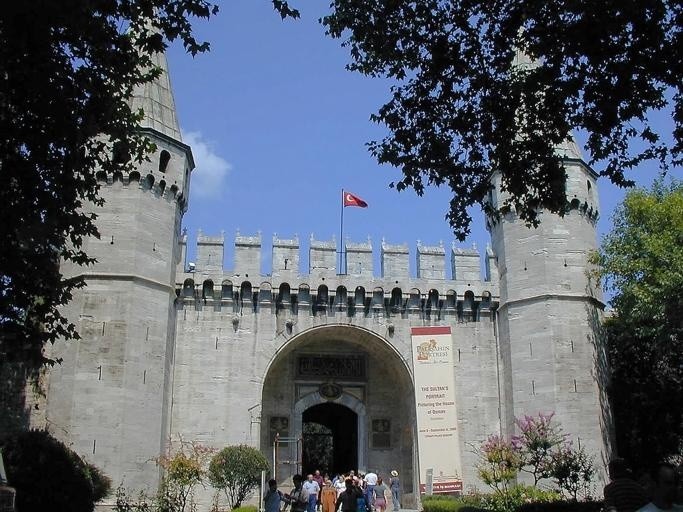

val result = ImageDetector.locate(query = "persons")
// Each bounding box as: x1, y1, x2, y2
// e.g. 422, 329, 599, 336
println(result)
262, 478, 291, 512
388, 470, 401, 512
279, 469, 387, 512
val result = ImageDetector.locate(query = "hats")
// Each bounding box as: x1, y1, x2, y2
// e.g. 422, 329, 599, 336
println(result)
391, 470, 398, 476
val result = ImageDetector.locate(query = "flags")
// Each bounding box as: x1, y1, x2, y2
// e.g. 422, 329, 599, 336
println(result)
341, 188, 369, 211
